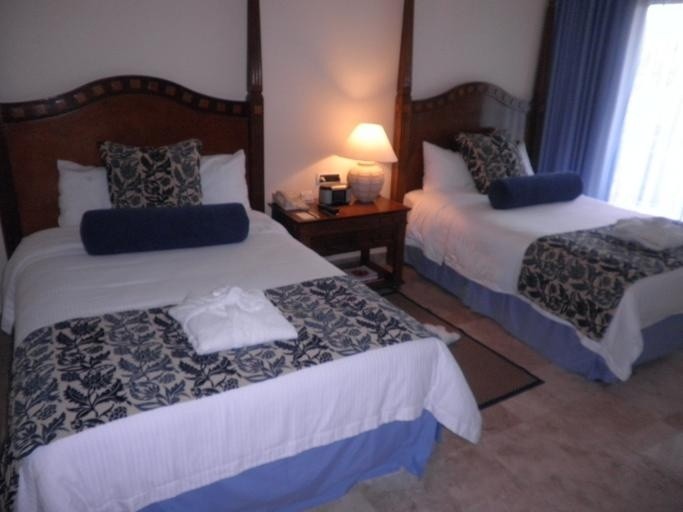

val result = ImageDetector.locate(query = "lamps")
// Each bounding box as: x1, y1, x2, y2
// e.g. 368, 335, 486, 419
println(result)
335, 120, 400, 208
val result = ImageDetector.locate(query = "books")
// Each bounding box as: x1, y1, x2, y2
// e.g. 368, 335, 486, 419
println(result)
343, 264, 384, 282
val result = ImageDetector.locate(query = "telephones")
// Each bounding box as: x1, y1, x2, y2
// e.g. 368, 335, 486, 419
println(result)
272, 190, 310, 211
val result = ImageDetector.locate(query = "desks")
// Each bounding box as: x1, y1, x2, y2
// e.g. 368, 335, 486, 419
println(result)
267, 188, 409, 276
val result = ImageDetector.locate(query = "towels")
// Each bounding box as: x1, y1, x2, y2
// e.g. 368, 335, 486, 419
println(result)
608, 214, 682, 255
166, 287, 295, 352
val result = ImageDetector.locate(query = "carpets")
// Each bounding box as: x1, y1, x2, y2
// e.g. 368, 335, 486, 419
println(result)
336, 261, 547, 418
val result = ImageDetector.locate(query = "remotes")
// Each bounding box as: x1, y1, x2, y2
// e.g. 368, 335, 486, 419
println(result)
319, 203, 339, 214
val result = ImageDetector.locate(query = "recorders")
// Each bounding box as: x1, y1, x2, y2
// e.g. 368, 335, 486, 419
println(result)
319, 184, 351, 205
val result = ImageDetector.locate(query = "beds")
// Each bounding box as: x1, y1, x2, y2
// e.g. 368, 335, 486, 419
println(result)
385, 0, 680, 383
1, 0, 485, 511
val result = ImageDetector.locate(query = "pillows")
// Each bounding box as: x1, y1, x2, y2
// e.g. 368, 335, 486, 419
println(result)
457, 123, 528, 195
79, 196, 254, 257
96, 135, 206, 209
417, 137, 533, 198
486, 167, 583, 207
52, 149, 251, 226
